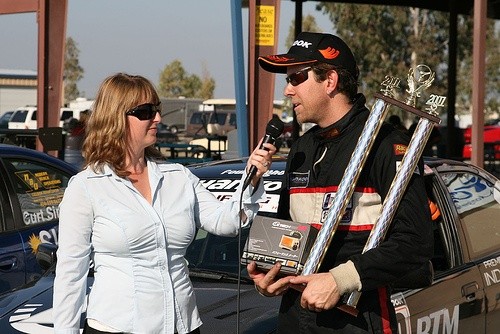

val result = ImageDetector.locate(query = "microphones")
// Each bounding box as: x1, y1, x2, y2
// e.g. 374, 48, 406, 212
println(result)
242, 117, 285, 191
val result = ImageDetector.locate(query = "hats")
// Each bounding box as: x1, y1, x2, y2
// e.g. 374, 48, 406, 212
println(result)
257, 31, 360, 77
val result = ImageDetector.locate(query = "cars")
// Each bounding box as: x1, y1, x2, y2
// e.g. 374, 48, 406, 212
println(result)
0, 154, 500, 334
0, 144, 81, 293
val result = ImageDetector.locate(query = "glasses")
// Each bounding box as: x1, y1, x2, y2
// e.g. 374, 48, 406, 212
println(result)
126, 100, 163, 122
285, 67, 314, 87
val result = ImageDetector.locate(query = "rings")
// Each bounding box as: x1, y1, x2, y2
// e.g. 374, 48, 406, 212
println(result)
264, 161, 270, 167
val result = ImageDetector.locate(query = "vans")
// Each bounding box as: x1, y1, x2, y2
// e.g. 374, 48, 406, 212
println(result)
8, 106, 75, 129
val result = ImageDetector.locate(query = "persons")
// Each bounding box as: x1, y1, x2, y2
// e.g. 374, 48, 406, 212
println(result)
247, 32, 436, 334
389, 116, 408, 135
53, 72, 277, 334
